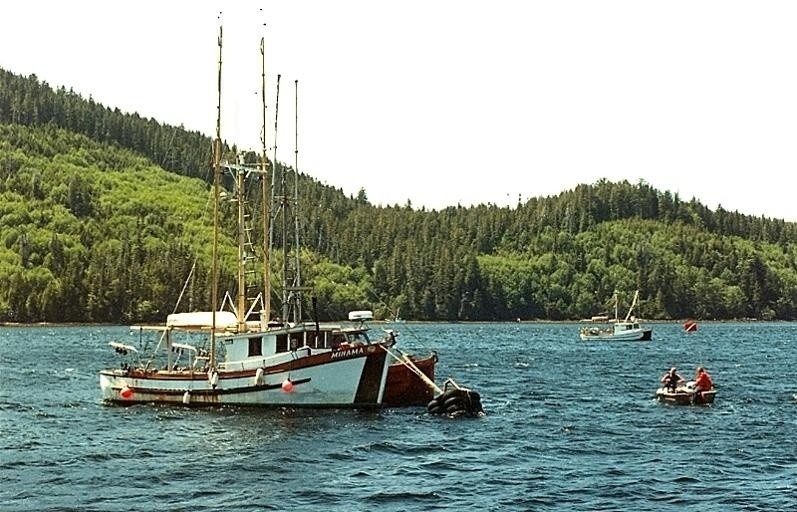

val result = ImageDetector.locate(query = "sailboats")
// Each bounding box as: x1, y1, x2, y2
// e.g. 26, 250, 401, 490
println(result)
578, 289, 653, 341
98, 22, 396, 410
268, 75, 439, 404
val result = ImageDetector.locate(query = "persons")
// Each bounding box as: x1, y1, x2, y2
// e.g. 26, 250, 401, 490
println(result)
291, 330, 342, 349
660, 367, 684, 393
692, 367, 712, 390
581, 325, 612, 336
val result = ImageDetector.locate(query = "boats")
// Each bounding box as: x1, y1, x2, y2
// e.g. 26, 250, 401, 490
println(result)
655, 379, 717, 405
